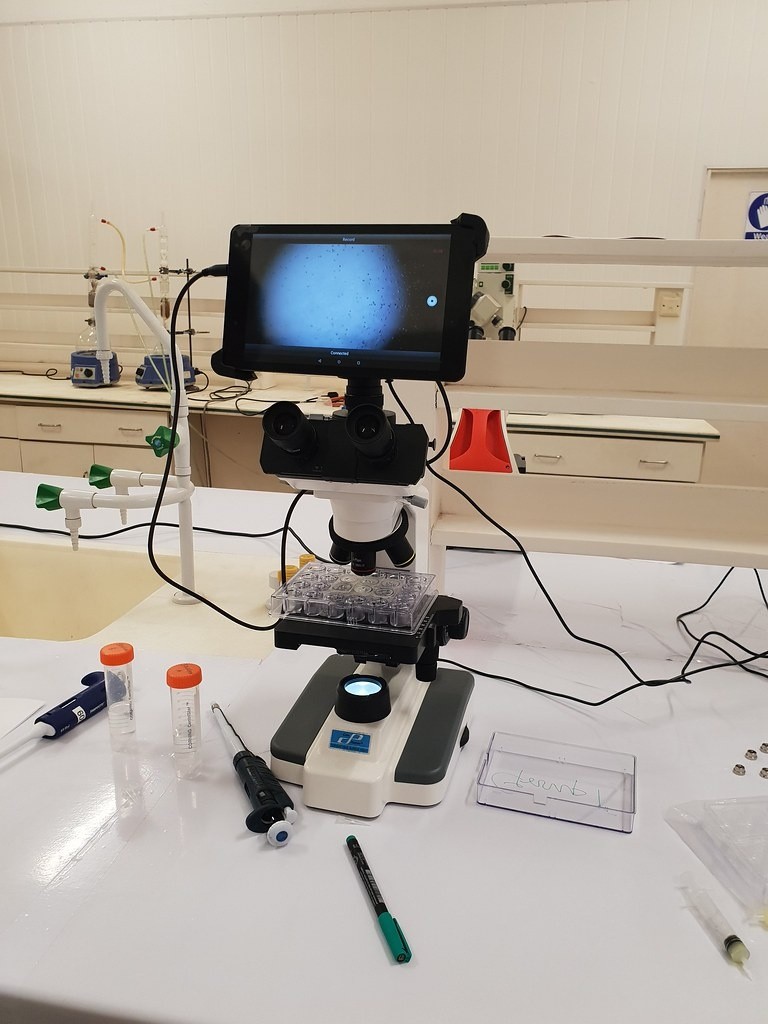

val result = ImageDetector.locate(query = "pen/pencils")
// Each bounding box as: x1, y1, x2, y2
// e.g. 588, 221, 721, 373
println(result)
347, 835, 412, 966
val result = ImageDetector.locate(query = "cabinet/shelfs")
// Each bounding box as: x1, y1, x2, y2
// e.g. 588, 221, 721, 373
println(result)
507, 412, 721, 484
0, 375, 204, 485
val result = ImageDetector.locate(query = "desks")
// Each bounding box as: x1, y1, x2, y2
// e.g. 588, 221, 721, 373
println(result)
0, 471, 768, 1024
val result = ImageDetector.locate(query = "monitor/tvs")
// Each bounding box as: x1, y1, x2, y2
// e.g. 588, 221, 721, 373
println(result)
221, 224, 475, 382
